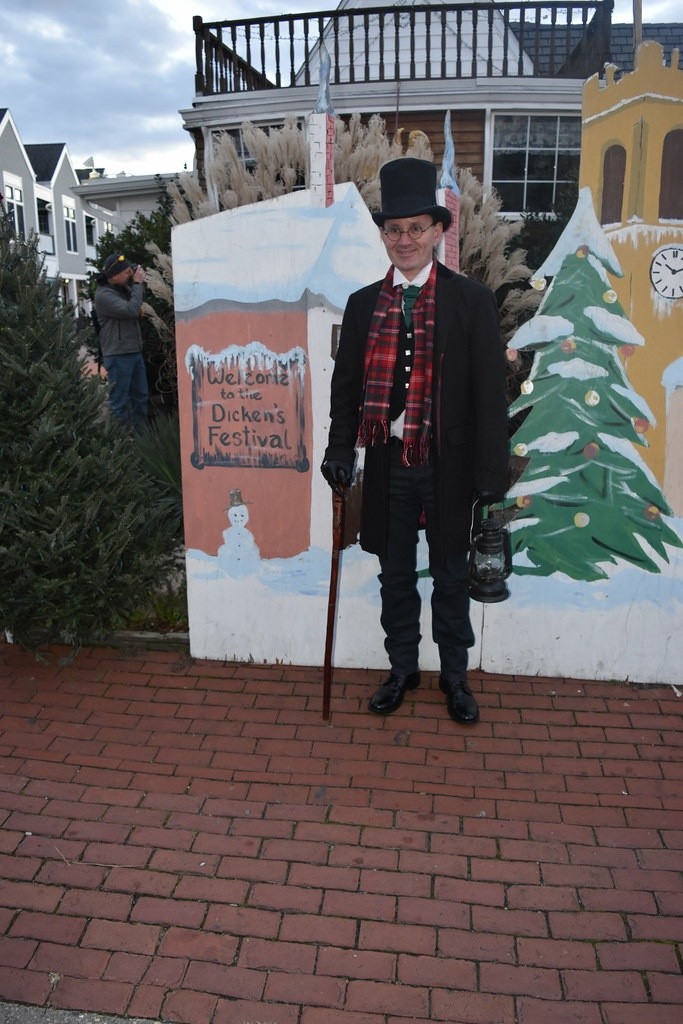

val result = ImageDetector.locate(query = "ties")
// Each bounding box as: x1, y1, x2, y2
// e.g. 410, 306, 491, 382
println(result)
402, 285, 424, 327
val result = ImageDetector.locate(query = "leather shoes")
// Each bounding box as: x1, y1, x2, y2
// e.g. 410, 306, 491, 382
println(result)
367, 673, 420, 715
439, 675, 480, 724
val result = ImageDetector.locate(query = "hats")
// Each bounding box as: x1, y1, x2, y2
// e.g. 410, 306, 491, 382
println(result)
102, 254, 130, 278
372, 158, 453, 232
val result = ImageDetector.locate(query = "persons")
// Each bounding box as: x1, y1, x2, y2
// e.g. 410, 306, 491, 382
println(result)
321, 155, 512, 724
94, 253, 149, 436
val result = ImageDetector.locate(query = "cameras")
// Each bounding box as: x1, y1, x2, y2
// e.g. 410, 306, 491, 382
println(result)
130, 262, 146, 275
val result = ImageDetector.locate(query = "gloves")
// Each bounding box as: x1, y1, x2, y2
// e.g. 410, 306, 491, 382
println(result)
321, 460, 353, 496
476, 489, 505, 508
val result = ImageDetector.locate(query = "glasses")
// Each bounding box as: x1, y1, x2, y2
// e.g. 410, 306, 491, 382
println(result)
381, 222, 436, 242
107, 254, 125, 272
